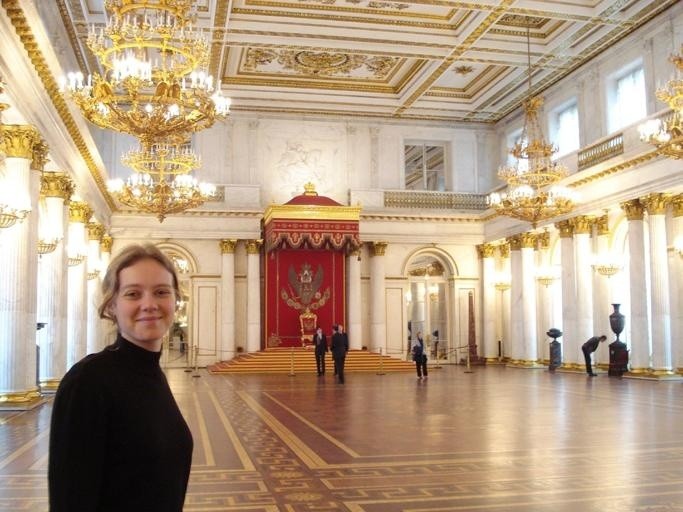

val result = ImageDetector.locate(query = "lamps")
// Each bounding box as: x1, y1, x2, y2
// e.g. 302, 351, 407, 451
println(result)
100, 173, 222, 223
115, 137, 198, 178
51, 66, 235, 147
482, 13, 578, 231
71, 1, 208, 79
637, 44, 683, 162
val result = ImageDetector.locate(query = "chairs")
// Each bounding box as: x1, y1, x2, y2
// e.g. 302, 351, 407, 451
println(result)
297, 308, 321, 348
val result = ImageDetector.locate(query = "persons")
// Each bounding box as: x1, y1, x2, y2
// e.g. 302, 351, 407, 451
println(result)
313, 323, 349, 384
49, 244, 194, 512
582, 335, 607, 376
411, 332, 428, 378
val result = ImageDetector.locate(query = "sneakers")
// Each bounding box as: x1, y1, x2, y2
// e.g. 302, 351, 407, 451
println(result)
587, 372, 598, 377
415, 375, 429, 382
